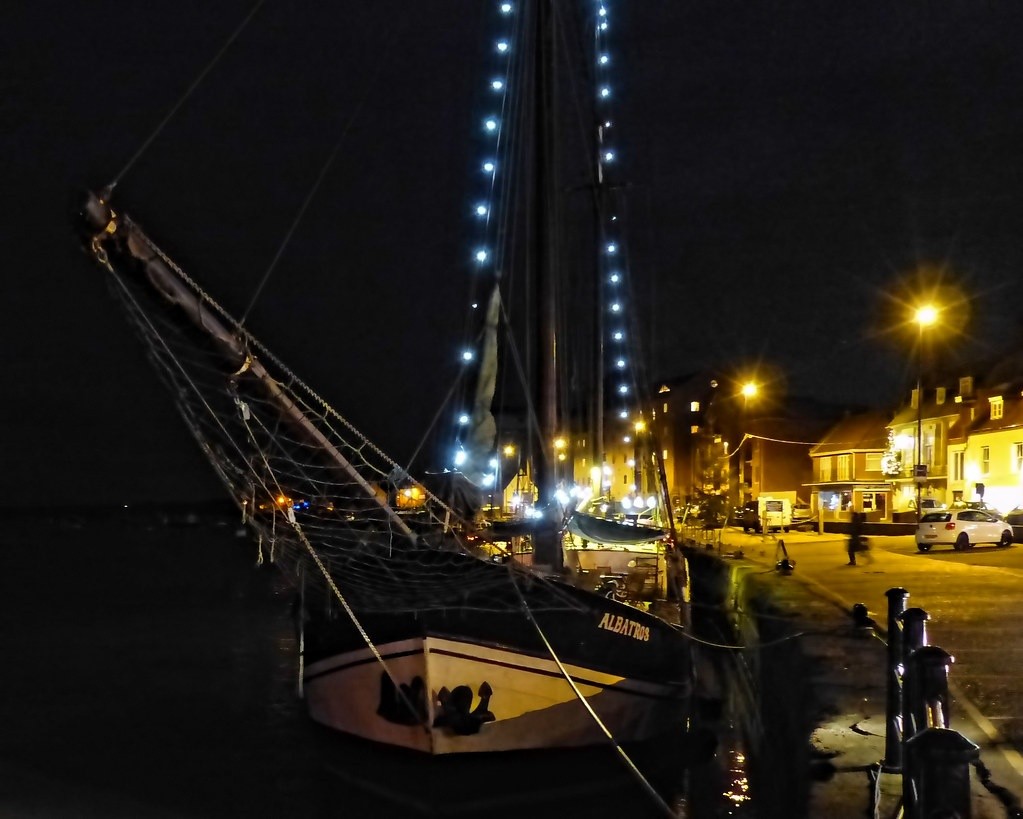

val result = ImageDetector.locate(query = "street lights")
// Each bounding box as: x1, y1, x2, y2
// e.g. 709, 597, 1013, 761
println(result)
742, 384, 757, 433
915, 309, 937, 522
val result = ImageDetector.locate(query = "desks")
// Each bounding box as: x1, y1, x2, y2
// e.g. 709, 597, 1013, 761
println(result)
612, 572, 628, 576
600, 575, 624, 579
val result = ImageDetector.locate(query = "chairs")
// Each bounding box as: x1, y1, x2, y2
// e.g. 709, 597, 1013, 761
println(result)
597, 566, 611, 576
635, 556, 659, 583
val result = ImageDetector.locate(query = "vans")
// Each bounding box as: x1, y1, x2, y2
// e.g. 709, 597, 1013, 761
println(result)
742, 497, 793, 533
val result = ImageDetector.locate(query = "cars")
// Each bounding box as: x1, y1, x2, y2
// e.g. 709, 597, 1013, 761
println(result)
914, 508, 1014, 551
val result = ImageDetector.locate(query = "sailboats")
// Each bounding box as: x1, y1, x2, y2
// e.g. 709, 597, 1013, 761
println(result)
77, 0, 700, 819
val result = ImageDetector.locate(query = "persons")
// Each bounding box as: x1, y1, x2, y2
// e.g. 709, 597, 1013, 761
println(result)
416, 536, 430, 549
437, 531, 463, 552
844, 508, 869, 566
876, 494, 885, 518
949, 494, 966, 509
